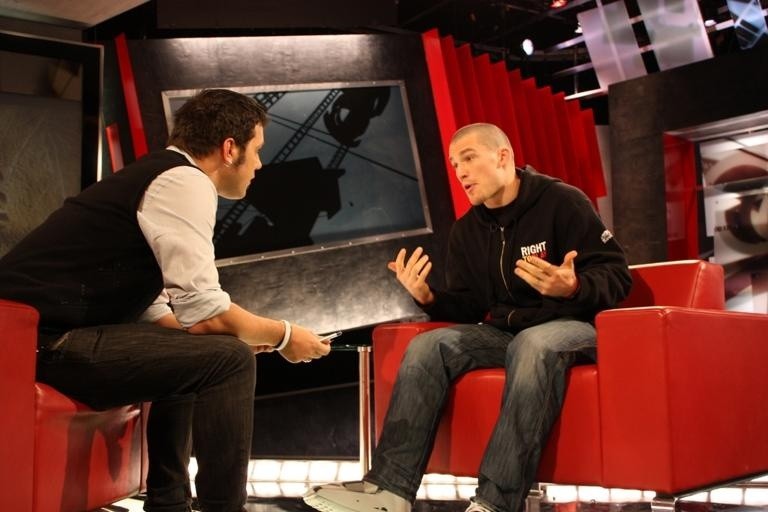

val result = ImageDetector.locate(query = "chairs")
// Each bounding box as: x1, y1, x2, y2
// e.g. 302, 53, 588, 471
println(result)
1, 299, 152, 511
373, 260, 768, 511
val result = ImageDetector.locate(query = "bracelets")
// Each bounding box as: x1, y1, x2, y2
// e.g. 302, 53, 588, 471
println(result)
272, 318, 291, 351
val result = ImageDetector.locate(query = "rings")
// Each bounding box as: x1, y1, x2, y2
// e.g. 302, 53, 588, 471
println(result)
304, 359, 312, 363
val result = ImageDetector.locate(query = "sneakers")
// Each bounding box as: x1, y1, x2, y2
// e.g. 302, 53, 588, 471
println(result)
303, 479, 412, 512
465, 500, 491, 512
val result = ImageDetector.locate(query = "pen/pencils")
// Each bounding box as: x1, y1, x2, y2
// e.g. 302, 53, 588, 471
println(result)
321, 332, 343, 342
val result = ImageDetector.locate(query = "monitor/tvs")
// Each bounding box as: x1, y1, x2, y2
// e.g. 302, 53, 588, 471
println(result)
0, 29, 106, 263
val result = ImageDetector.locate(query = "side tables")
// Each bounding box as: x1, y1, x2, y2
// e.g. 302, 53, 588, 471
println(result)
328, 342, 374, 482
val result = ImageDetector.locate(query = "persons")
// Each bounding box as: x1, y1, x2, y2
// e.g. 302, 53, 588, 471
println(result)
293, 122, 632, 512
0, 86, 333, 512
